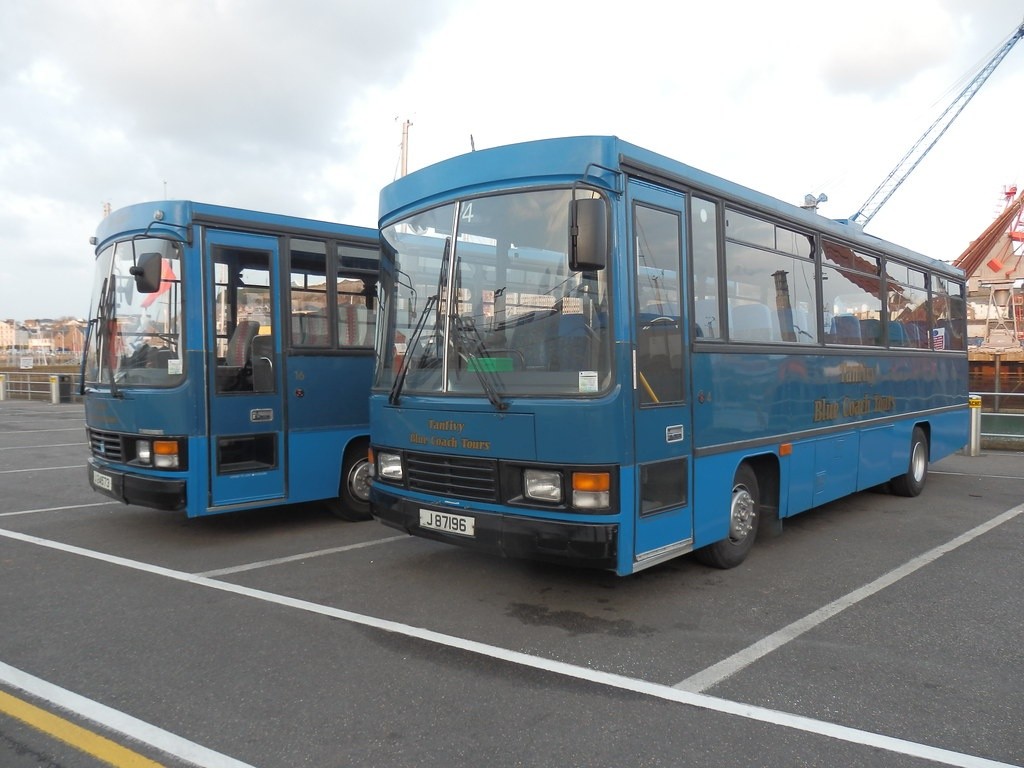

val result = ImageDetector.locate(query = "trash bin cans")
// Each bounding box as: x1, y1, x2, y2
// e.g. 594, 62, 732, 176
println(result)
48, 375, 72, 403
967, 395, 982, 458
0, 374, 7, 400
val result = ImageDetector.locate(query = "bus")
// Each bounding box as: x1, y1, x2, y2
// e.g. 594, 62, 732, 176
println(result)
78, 199, 736, 521
364, 134, 971, 578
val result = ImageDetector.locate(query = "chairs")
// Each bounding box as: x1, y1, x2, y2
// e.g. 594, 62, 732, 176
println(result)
290, 303, 377, 343
510, 311, 589, 368
219, 319, 261, 371
693, 300, 966, 348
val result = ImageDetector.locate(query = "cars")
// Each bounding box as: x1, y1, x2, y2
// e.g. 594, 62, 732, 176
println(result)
5, 347, 70, 356
